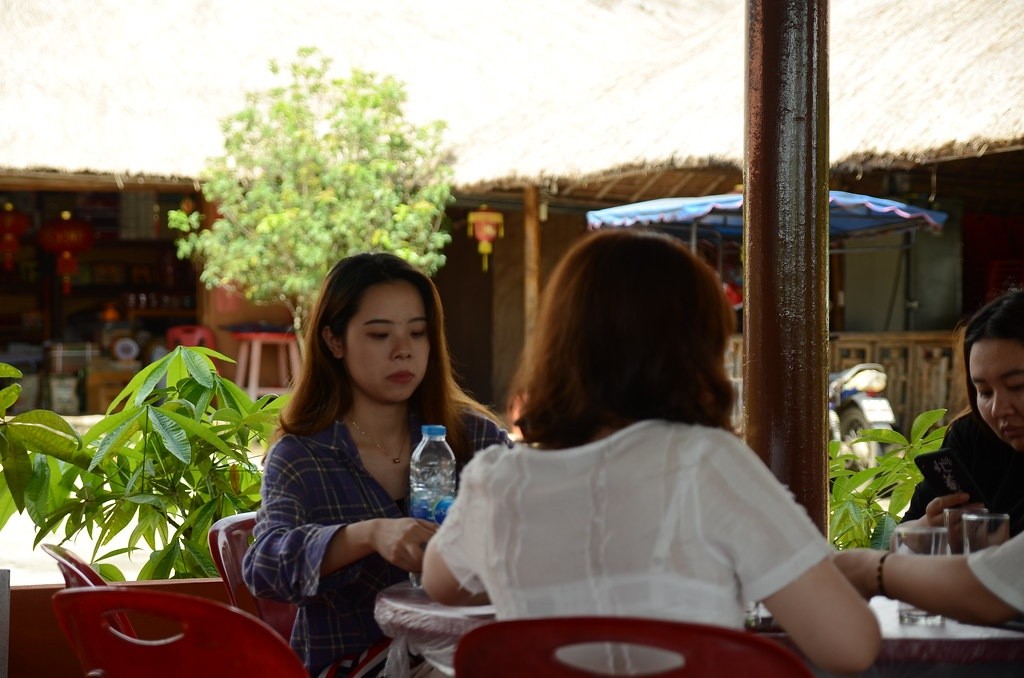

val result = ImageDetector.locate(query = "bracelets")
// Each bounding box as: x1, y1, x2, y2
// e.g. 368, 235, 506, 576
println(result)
876, 553, 890, 594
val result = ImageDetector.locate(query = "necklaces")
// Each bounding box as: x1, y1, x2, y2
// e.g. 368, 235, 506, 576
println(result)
350, 416, 409, 464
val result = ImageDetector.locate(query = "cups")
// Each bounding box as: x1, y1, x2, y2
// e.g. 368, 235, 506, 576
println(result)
896, 526, 948, 627
943, 509, 989, 556
961, 514, 1010, 555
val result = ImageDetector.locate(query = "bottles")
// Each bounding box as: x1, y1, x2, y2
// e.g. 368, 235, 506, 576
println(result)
409, 425, 457, 593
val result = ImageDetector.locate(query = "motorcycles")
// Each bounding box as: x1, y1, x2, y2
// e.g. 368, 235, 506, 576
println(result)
727, 333, 897, 495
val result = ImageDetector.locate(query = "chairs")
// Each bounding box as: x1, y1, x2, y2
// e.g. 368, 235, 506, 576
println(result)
51, 587, 310, 678
208, 511, 300, 644
40, 544, 138, 641
452, 615, 815, 678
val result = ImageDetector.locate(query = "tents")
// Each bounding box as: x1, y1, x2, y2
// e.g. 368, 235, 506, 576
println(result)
588, 184, 946, 331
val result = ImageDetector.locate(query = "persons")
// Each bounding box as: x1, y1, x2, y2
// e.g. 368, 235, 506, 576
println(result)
831, 289, 1024, 627
240, 253, 513, 678
423, 230, 879, 678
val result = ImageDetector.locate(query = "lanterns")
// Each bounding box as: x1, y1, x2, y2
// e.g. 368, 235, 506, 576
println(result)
40, 218, 94, 294
0, 213, 33, 269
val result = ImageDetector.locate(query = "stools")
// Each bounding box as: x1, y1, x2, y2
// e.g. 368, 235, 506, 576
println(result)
230, 333, 301, 404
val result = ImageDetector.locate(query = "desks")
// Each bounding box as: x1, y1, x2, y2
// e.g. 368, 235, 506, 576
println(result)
374, 576, 1024, 678
